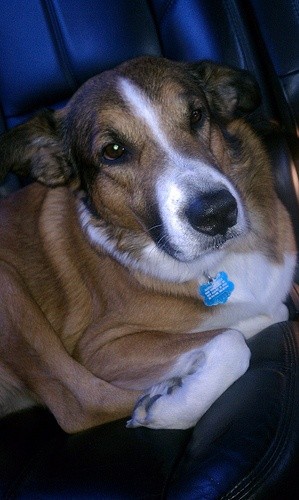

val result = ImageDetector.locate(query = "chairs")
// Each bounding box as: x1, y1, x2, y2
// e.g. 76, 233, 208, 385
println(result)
0, 1, 298, 499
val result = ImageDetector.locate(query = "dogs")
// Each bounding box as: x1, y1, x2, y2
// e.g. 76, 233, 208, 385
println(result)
1, 57, 298, 436
